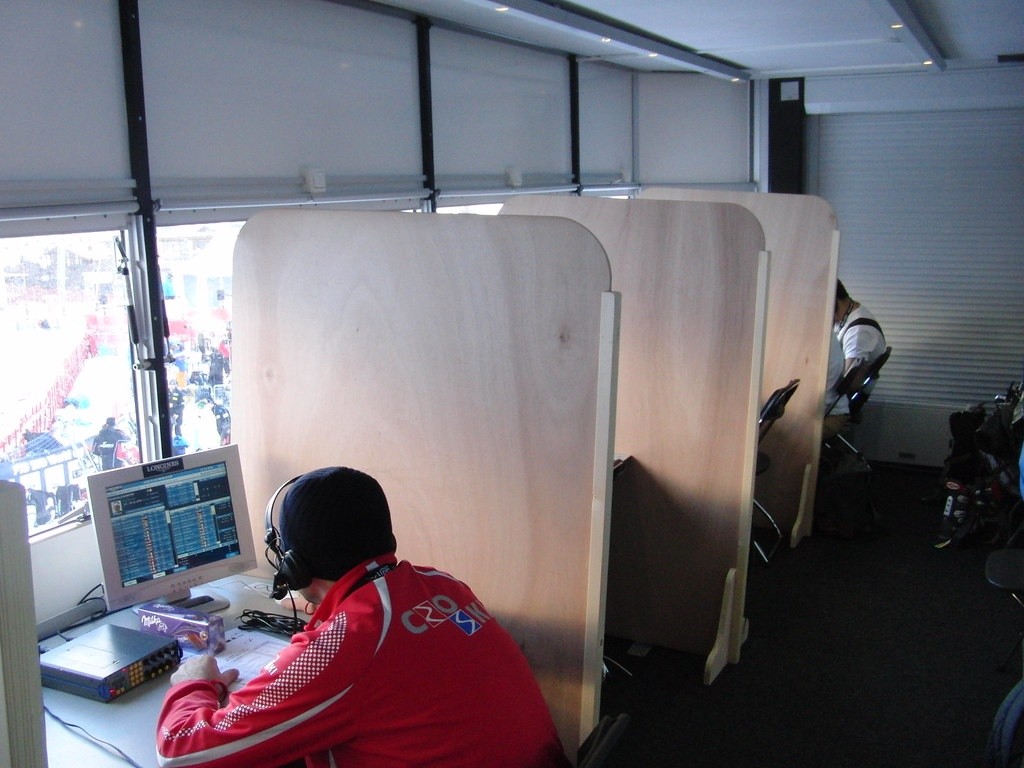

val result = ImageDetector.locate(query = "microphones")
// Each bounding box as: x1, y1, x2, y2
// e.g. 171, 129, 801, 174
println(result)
272, 585, 289, 600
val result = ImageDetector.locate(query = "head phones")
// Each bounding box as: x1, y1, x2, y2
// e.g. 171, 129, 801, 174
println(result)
263, 473, 310, 591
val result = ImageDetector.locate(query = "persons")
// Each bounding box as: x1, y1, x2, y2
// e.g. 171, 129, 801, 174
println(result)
16, 269, 231, 470
812, 280, 888, 440
155, 465, 573, 768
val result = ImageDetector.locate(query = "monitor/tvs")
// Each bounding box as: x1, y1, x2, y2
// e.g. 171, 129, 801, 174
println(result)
86, 445, 258, 615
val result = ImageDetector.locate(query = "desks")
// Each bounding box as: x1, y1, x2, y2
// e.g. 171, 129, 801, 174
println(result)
37, 568, 320, 768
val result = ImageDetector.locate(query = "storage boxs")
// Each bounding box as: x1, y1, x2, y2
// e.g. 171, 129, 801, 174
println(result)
137, 601, 229, 655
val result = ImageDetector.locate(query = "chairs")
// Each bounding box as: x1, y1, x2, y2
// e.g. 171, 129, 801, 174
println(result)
748, 378, 801, 566
822, 347, 892, 526
